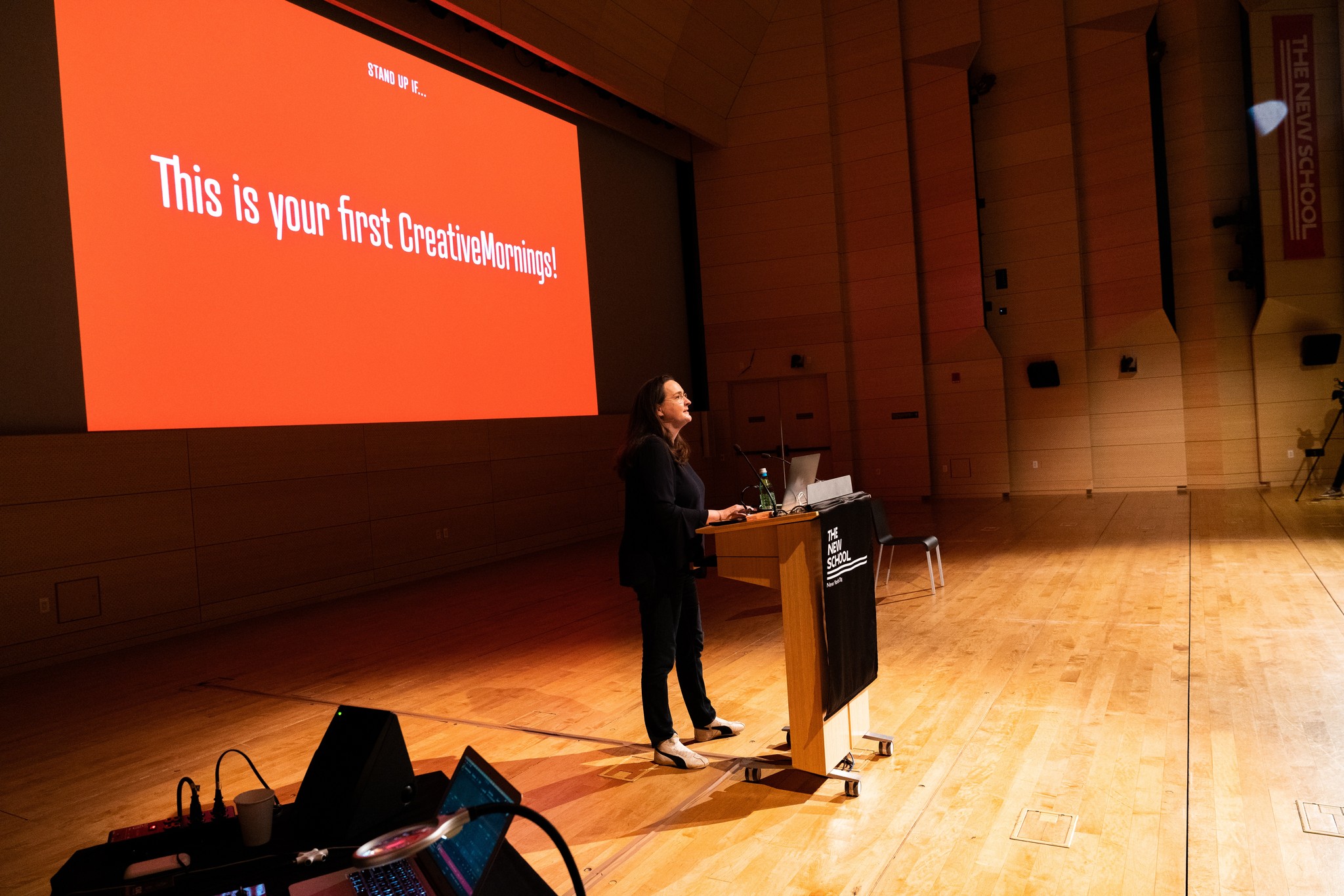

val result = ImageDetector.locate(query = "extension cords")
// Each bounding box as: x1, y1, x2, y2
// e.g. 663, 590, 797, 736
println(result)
109, 805, 235, 844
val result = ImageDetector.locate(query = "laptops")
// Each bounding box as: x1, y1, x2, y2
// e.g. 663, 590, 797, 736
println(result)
287, 745, 522, 896
751, 453, 821, 513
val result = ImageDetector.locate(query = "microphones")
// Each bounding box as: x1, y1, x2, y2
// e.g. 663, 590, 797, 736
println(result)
760, 453, 825, 483
733, 443, 781, 517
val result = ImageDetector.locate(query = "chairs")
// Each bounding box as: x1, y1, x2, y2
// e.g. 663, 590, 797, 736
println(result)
871, 502, 945, 595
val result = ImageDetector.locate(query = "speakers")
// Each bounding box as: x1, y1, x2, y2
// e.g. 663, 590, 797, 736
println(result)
1026, 361, 1060, 389
1303, 334, 1342, 365
295, 706, 414, 802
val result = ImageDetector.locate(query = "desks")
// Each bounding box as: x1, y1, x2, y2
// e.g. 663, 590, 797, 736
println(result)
695, 490, 892, 783
46, 771, 558, 896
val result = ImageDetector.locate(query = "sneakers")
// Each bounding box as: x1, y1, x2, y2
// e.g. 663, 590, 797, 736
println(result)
693, 715, 745, 742
653, 732, 709, 771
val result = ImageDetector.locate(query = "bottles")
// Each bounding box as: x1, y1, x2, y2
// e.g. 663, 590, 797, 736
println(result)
759, 468, 777, 510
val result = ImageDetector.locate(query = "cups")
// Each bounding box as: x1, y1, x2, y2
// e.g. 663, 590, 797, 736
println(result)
234, 787, 276, 845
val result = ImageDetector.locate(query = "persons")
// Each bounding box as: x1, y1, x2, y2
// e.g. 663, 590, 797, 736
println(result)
616, 367, 757, 773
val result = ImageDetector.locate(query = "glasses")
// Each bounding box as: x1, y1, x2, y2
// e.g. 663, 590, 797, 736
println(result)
660, 391, 689, 403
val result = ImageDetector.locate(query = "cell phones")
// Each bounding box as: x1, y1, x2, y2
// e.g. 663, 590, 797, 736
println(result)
214, 882, 267, 896
709, 517, 747, 527
